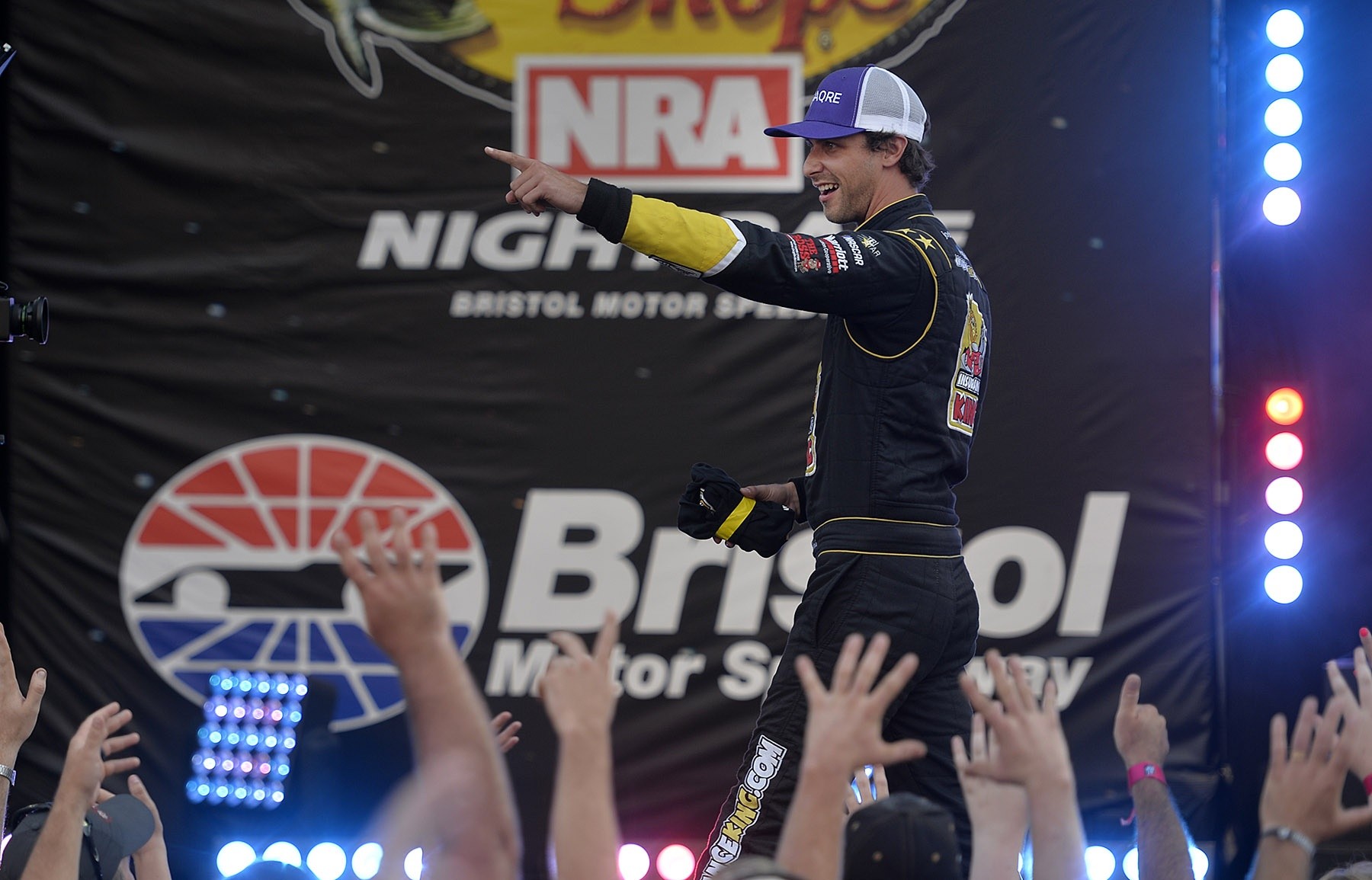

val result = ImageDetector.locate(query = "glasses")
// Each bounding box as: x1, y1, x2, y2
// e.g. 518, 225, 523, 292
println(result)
11, 803, 105, 880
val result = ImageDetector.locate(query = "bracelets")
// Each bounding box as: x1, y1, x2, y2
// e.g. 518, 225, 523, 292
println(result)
1261, 825, 1317, 857
1362, 773, 1372, 796
1128, 762, 1165, 791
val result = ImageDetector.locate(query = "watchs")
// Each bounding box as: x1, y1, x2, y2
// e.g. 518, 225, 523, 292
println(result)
0, 764, 16, 787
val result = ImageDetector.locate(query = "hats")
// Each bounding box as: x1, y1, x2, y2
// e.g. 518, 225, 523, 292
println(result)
0, 793, 155, 880
842, 793, 962, 880
764, 64, 928, 144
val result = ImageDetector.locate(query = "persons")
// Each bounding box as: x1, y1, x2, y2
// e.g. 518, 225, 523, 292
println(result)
0, 506, 1372, 880
481, 63, 993, 880
